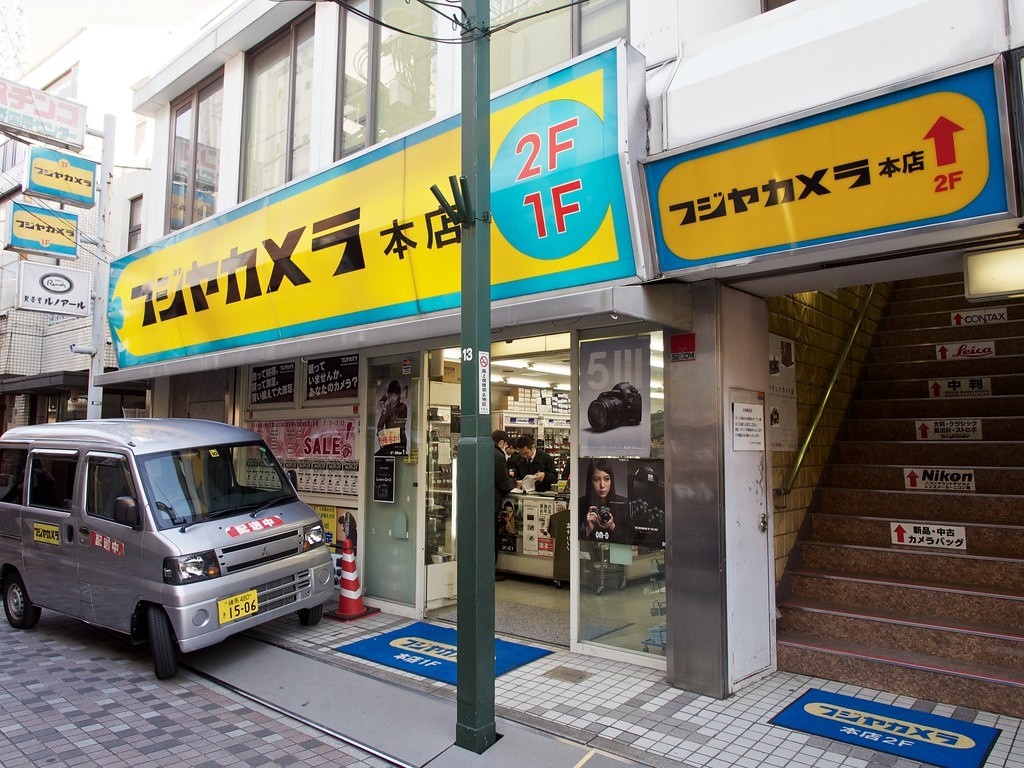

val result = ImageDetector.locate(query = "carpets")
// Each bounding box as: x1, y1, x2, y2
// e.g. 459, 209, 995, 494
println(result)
332, 621, 555, 686
767, 687, 1003, 768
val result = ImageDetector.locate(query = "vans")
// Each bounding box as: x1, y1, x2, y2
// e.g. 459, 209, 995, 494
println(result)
0, 417, 336, 681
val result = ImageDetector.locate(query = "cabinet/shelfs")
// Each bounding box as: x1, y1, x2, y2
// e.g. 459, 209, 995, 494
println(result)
490, 411, 665, 589
428, 404, 459, 604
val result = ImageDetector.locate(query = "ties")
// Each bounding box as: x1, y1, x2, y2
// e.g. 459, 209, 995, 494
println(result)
528, 458, 532, 467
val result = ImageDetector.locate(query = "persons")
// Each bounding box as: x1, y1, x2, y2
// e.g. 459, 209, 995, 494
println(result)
578, 458, 633, 544
496, 501, 515, 533
373, 380, 407, 456
489, 430, 570, 582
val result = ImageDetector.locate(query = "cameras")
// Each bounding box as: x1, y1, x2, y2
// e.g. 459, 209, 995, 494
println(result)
587, 382, 642, 432
595, 507, 610, 523
501, 511, 509, 517
648, 506, 664, 523
380, 394, 388, 403
629, 497, 648, 521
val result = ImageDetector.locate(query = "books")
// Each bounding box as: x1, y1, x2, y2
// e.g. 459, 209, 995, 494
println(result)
510, 474, 538, 494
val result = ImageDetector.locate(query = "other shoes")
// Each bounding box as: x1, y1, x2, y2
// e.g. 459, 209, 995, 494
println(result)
495, 575, 506, 582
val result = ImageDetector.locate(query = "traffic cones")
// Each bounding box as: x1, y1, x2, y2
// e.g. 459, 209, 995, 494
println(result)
321, 538, 381, 623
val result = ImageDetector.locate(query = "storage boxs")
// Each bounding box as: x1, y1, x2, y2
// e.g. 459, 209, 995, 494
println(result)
499, 387, 571, 412
